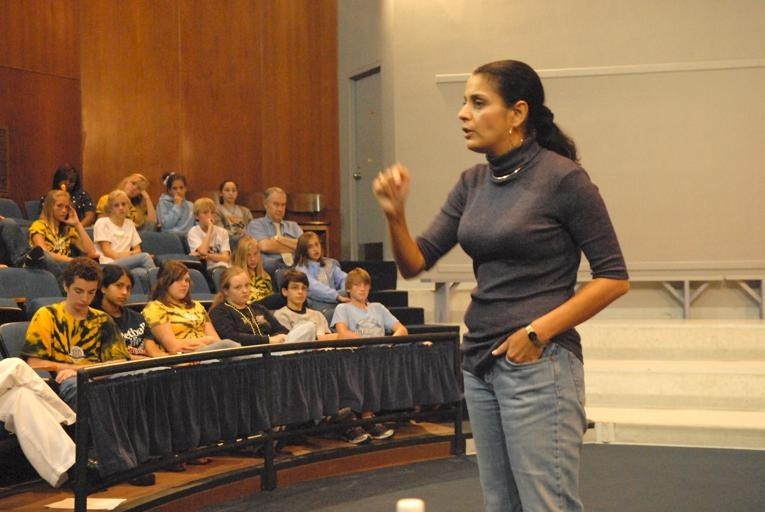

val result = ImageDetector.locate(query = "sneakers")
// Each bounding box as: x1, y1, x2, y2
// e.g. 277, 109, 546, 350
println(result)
363, 416, 395, 439
341, 418, 372, 444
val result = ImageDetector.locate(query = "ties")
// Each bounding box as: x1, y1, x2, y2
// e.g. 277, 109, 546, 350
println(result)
275, 222, 294, 267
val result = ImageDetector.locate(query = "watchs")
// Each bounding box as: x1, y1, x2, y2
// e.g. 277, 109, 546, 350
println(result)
524, 324, 548, 351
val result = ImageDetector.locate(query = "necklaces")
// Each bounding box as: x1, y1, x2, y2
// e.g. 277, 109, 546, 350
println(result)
489, 160, 523, 184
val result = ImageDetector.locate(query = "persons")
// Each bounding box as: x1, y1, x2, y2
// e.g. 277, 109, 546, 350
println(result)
371, 57, 635, 512
0, 356, 155, 492
187, 181, 303, 303
156, 171, 195, 236
97, 172, 157, 229
30, 163, 100, 262
21, 268, 421, 472
93, 189, 157, 295
291, 230, 352, 325
